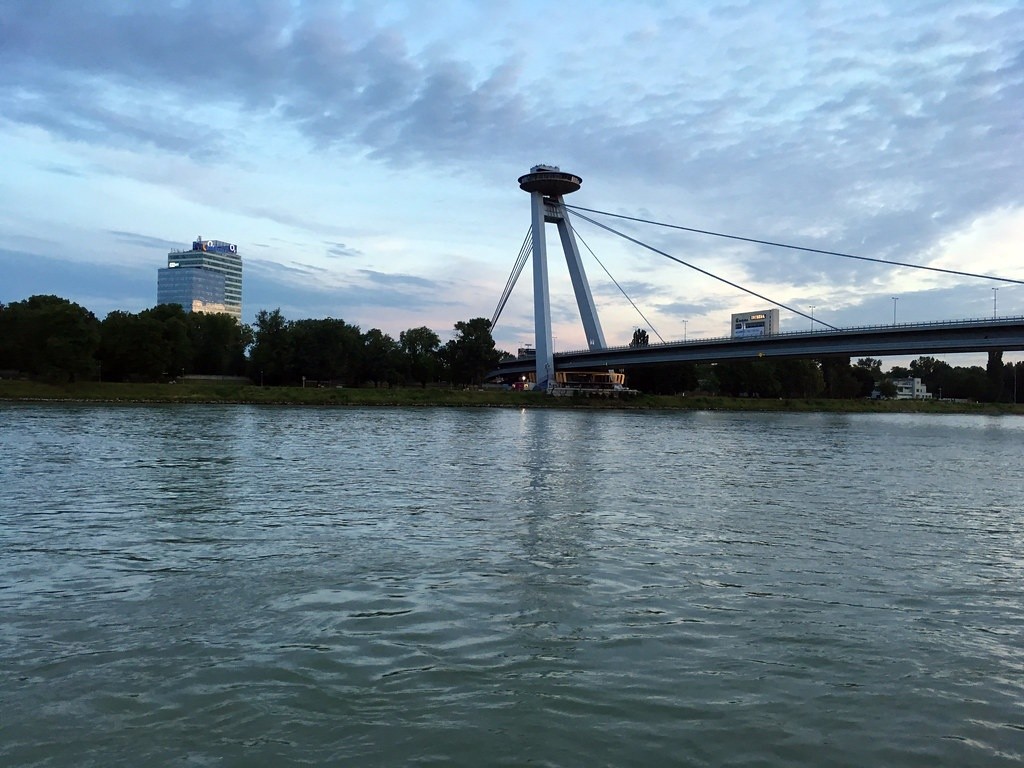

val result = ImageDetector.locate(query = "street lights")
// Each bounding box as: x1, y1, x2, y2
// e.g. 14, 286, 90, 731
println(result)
892, 296, 899, 325
682, 320, 689, 343
991, 287, 1000, 319
551, 336, 557, 354
809, 305, 816, 332
